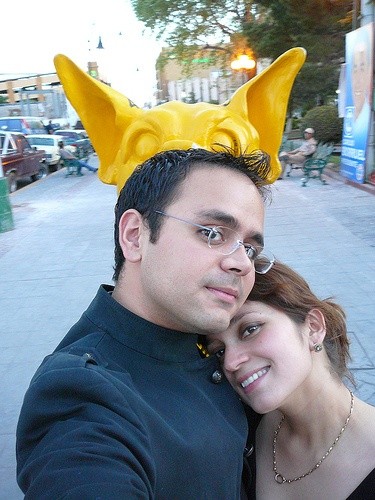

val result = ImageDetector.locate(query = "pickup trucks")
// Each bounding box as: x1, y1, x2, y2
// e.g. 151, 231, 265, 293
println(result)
0, 134, 46, 194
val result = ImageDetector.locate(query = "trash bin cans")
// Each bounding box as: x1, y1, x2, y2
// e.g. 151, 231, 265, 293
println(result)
0, 177, 14, 233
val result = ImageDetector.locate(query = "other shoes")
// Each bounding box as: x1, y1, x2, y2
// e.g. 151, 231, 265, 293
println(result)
278, 178, 282, 180
279, 155, 289, 161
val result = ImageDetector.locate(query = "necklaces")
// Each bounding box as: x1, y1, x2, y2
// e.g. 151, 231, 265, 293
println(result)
273, 386, 354, 484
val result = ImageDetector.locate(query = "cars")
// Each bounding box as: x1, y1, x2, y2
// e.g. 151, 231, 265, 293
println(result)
24, 121, 94, 172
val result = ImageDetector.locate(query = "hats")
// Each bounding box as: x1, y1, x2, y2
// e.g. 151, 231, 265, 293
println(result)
304, 127, 314, 134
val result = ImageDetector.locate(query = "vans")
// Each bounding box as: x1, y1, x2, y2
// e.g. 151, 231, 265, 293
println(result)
0, 116, 53, 135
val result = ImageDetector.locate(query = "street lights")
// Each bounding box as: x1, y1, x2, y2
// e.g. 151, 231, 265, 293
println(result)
230, 55, 255, 86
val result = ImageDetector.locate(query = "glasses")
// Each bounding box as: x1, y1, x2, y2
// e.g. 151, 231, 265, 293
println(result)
154, 209, 276, 275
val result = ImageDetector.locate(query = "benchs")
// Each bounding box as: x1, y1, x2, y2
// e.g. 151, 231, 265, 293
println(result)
57, 150, 91, 177
283, 139, 335, 187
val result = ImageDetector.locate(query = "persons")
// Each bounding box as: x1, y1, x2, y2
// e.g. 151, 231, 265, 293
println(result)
47, 120, 53, 134
348, 27, 370, 134
208, 258, 375, 500
16, 137, 279, 500
277, 128, 316, 180
58, 140, 98, 177
